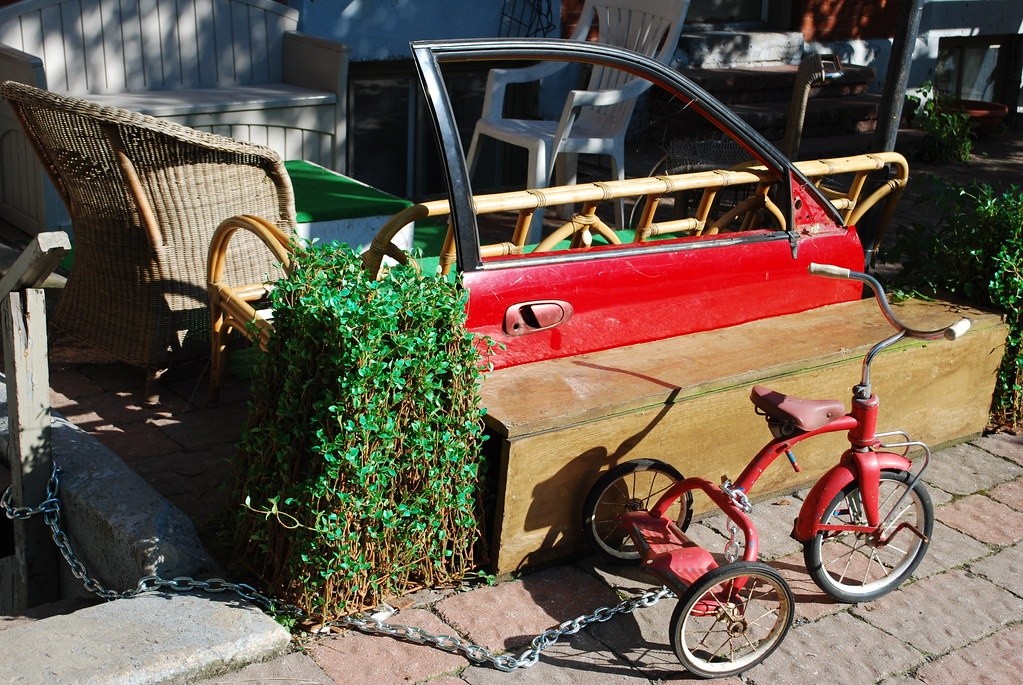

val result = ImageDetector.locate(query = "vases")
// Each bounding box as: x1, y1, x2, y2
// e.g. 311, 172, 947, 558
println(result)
939, 99, 1009, 134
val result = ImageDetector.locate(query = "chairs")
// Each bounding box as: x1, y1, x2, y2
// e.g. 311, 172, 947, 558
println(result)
0, 80, 300, 400
447, 0, 691, 246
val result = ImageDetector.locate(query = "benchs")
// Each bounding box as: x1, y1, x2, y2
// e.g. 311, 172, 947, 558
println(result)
468, 290, 1010, 575
206, 148, 910, 403
0, 0, 353, 238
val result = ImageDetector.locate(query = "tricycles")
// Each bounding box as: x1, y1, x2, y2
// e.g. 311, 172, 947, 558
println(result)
582, 259, 970, 680
